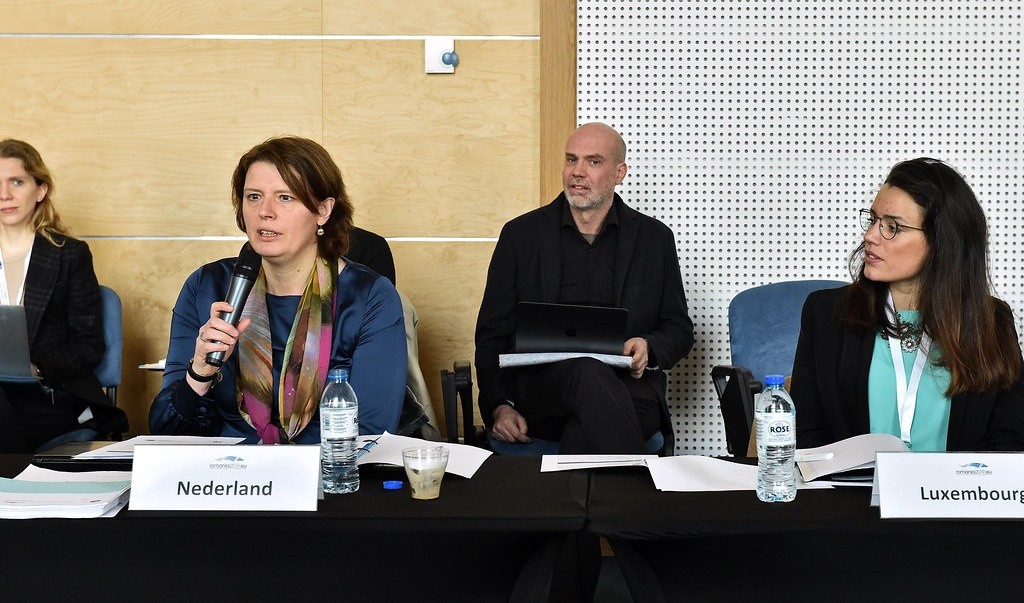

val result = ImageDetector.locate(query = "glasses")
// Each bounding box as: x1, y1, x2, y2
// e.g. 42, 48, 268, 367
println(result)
860, 207, 924, 241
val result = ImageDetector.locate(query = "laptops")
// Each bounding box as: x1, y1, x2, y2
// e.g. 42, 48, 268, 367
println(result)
515, 301, 628, 356
33, 441, 133, 465
0, 305, 32, 377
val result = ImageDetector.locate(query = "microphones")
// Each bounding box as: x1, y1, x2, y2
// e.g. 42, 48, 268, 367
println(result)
205, 240, 262, 367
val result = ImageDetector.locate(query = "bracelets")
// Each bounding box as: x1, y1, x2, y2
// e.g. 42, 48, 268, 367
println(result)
34, 365, 40, 373
188, 358, 218, 382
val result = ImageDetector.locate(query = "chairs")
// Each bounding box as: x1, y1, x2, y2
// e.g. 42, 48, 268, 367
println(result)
440, 359, 667, 454
711, 280, 850, 458
346, 226, 431, 438
100, 286, 125, 443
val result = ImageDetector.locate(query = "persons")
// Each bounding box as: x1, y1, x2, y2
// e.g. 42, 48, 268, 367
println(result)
147, 137, 408, 449
475, 122, 695, 454
0, 139, 128, 455
792, 157, 1024, 451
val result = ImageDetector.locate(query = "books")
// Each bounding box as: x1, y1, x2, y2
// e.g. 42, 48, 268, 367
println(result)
796, 434, 912, 486
358, 430, 493, 480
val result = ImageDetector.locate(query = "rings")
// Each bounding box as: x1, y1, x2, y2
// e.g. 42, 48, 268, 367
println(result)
200, 332, 206, 342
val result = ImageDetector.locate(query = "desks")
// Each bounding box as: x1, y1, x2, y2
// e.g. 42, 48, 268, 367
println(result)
585, 465, 1023, 603
0, 452, 587, 603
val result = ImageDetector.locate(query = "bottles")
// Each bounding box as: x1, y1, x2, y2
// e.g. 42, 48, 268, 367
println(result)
754, 374, 797, 502
319, 369, 361, 493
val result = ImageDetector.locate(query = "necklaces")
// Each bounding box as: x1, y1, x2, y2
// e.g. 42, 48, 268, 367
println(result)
896, 312, 923, 352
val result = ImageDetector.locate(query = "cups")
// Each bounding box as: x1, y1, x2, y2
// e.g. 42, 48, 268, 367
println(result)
402, 447, 449, 499
